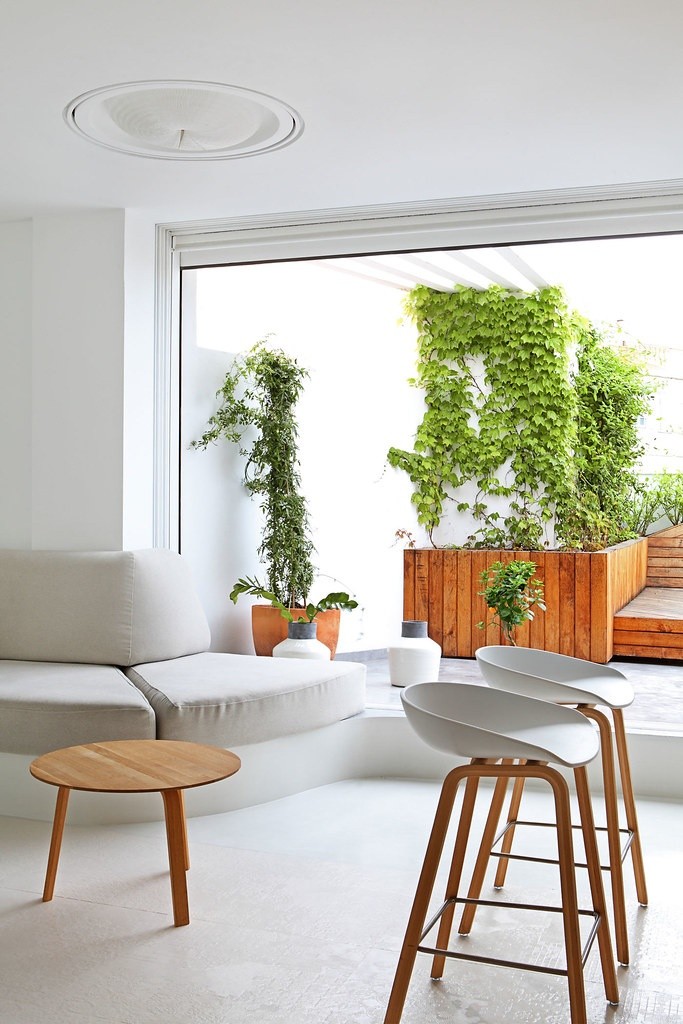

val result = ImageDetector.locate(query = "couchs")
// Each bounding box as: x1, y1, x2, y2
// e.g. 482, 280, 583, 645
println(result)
0, 545, 368, 756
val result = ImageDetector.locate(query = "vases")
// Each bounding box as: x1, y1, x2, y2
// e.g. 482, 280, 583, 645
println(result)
385, 621, 442, 688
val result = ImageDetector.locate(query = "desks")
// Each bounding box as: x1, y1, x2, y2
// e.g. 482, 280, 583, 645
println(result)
29, 740, 243, 928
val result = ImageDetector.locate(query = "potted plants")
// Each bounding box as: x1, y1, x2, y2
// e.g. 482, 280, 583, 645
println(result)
228, 573, 358, 663
183, 333, 365, 661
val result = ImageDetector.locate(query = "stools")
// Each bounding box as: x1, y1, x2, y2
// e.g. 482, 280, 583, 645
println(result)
457, 645, 649, 968
382, 682, 620, 1024
